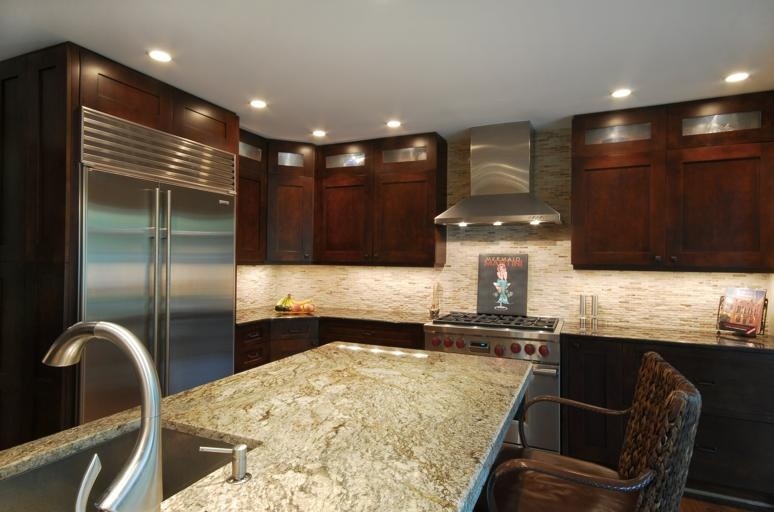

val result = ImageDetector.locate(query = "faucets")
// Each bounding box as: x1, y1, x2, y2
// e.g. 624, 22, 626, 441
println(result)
40, 319, 166, 512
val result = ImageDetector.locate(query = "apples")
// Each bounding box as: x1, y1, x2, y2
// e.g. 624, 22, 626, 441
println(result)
291, 304, 300, 312
303, 304, 313, 312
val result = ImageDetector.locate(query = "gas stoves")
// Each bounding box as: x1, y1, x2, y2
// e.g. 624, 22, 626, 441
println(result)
427, 309, 560, 359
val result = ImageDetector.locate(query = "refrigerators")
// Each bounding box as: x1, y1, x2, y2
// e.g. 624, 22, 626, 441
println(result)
76, 108, 240, 425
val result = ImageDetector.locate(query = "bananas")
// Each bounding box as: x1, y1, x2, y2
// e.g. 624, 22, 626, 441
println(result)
275, 293, 299, 310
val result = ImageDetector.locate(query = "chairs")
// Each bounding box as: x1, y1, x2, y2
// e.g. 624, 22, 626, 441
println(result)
483, 350, 702, 512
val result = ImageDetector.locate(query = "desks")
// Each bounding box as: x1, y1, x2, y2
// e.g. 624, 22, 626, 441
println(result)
0, 338, 534, 510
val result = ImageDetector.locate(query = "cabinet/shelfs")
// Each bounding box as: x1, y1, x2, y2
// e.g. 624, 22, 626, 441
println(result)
322, 316, 423, 351
570, 88, 774, 272
0, 40, 240, 156
236, 127, 315, 265
234, 317, 275, 374
314, 130, 447, 270
560, 331, 623, 471
274, 318, 320, 366
624, 332, 774, 511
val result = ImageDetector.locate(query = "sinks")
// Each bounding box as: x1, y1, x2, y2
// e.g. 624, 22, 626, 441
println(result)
0, 419, 264, 512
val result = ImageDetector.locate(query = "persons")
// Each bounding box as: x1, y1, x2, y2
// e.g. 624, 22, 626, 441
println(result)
497, 264, 510, 304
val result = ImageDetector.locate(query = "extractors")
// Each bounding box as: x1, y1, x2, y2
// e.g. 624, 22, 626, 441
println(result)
433, 120, 565, 227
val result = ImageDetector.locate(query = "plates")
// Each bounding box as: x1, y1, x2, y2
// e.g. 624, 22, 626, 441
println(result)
271, 310, 311, 315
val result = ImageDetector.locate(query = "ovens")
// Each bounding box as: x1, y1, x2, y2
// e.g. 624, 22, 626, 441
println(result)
503, 365, 560, 451
